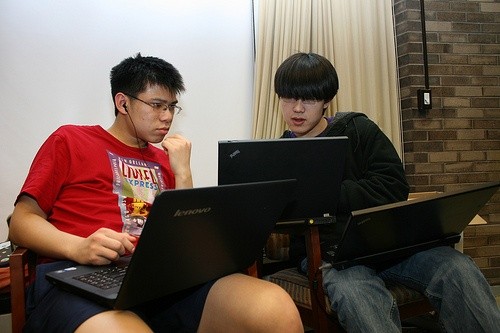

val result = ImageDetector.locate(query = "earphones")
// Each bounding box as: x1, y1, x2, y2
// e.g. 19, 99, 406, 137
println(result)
123, 102, 128, 112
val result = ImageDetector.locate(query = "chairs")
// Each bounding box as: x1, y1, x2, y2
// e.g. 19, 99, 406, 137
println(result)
248, 214, 456, 332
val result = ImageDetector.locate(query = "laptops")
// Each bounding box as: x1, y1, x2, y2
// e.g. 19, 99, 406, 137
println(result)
218, 136, 349, 222
45, 179, 297, 310
320, 180, 500, 271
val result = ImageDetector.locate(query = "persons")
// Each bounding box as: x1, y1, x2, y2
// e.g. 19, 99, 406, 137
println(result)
274, 52, 500, 333
9, 52, 304, 333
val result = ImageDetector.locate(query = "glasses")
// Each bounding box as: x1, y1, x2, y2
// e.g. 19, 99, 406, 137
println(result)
279, 96, 317, 105
135, 97, 182, 116
